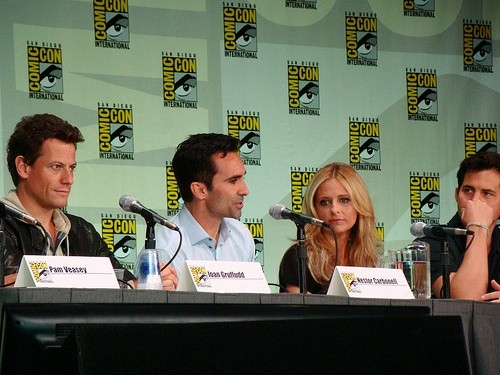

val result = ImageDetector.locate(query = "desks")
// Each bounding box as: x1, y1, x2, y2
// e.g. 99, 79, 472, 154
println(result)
0, 287, 500, 375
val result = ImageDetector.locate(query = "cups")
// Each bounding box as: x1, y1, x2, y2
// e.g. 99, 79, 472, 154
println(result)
137, 250, 162, 290
113, 269, 125, 289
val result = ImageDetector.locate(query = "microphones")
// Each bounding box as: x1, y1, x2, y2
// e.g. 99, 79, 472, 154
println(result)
119, 194, 179, 231
410, 222, 474, 238
269, 204, 330, 228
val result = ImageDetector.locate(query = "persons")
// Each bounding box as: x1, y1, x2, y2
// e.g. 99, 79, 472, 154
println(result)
132, 132, 256, 293
279, 162, 381, 296
0, 113, 180, 292
393, 150, 500, 304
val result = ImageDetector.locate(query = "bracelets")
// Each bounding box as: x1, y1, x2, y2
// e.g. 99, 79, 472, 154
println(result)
466, 222, 489, 234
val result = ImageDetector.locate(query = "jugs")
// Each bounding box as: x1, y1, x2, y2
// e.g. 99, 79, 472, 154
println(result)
376, 242, 431, 299
